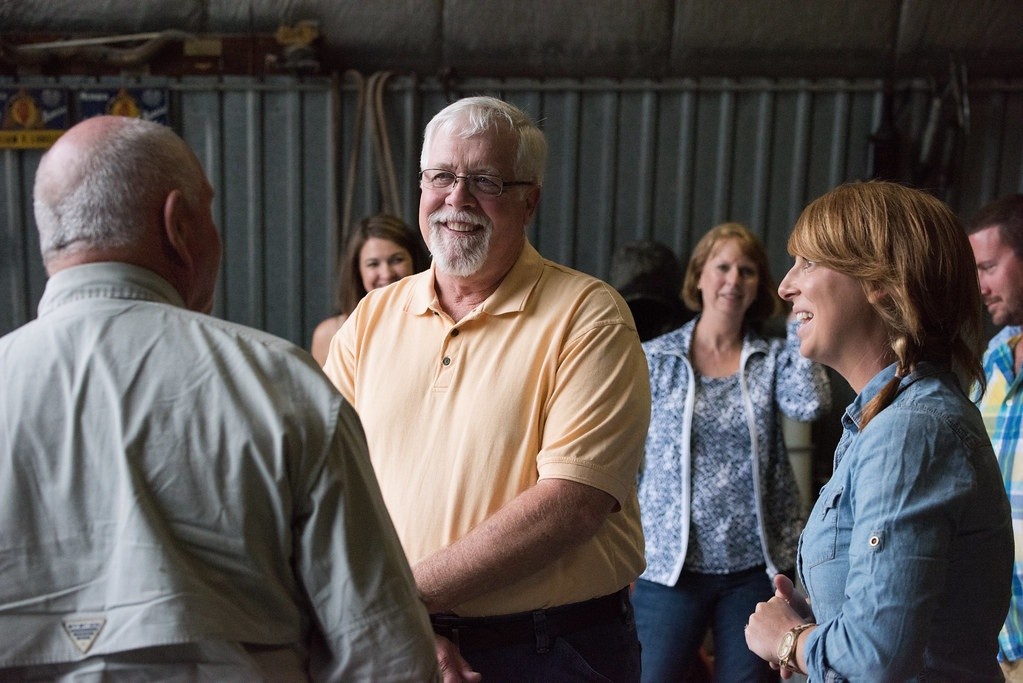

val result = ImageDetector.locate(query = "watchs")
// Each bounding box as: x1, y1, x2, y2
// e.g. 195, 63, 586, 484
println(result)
777, 624, 818, 676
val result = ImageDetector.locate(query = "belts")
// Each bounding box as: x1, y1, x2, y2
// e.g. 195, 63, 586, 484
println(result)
433, 587, 630, 649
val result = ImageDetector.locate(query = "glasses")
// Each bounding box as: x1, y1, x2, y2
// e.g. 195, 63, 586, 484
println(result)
418, 169, 537, 196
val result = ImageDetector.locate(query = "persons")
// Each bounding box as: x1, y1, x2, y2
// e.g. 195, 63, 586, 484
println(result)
965, 191, 1023, 683
741, 179, 1016, 683
325, 98, 650, 683
629, 221, 833, 682
610, 241, 693, 344
0, 115, 445, 683
313, 214, 431, 370
